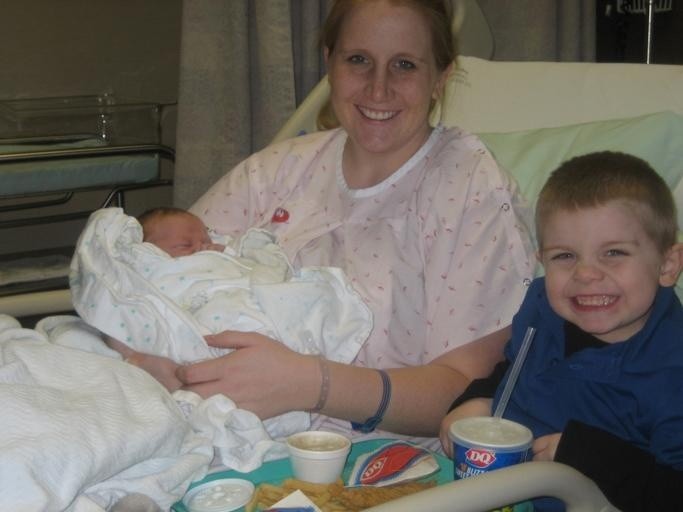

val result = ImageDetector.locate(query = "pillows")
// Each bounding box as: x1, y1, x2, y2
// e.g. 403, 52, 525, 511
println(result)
461, 112, 683, 300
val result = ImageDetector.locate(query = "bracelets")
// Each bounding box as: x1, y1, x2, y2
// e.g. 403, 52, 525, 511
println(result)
305, 349, 328, 416
351, 368, 392, 434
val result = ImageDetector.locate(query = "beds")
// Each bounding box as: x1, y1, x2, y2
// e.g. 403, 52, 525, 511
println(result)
0, 54, 683, 512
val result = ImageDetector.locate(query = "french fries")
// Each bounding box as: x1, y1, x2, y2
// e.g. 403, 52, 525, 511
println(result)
245, 478, 363, 512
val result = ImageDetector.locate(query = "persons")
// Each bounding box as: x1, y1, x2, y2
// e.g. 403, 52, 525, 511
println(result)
437, 150, 683, 512
97, 1, 539, 437
133, 206, 335, 430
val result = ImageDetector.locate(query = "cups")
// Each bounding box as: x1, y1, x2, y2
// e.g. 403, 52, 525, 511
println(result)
446, 415, 535, 512
284, 430, 352, 486
